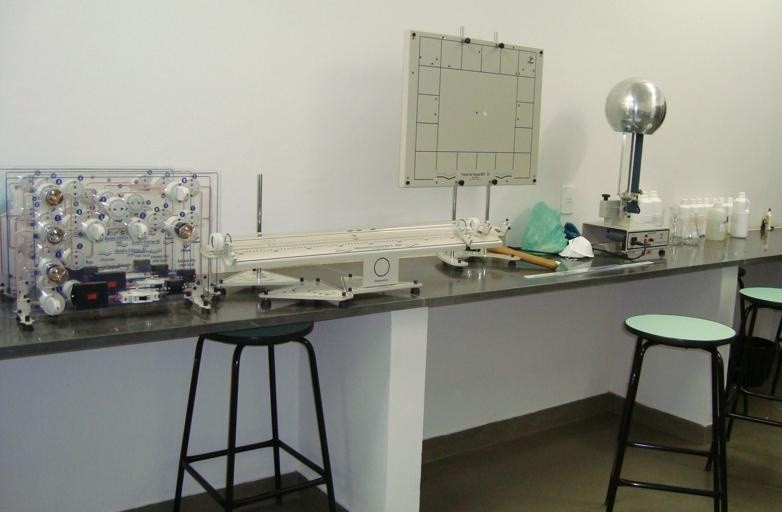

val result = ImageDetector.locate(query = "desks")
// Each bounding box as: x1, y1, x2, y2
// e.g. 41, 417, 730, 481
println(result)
0, 229, 782, 512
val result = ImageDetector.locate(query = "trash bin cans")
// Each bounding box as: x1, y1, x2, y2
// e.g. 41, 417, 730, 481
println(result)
727, 335, 779, 389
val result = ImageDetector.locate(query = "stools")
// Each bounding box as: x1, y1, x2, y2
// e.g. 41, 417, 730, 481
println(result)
705, 287, 782, 470
604, 315, 737, 512
171, 322, 337, 512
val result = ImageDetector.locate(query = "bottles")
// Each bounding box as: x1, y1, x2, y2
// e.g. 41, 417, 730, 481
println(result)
764, 208, 773, 231
637, 191, 751, 246
